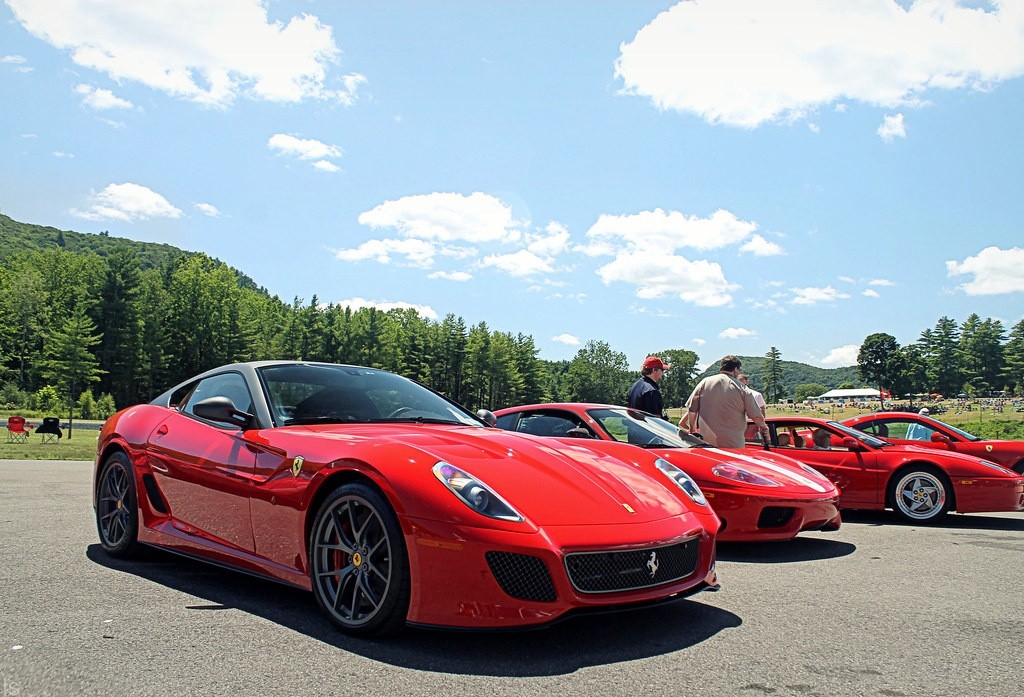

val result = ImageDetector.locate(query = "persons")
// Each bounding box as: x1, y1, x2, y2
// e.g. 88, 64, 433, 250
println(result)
905, 407, 931, 441
628, 357, 669, 445
812, 428, 832, 450
678, 354, 771, 447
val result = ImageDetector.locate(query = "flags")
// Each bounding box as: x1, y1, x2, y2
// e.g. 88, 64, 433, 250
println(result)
888, 390, 890, 397
880, 388, 886, 399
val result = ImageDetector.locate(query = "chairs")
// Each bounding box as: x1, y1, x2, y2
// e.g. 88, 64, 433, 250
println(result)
7, 417, 29, 444
878, 425, 889, 437
35, 417, 62, 444
794, 436, 805, 448
778, 433, 790, 447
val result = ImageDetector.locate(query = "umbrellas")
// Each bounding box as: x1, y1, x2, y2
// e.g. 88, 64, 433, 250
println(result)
958, 393, 966, 397
931, 393, 938, 401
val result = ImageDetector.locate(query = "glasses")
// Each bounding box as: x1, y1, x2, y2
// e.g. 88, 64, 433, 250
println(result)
737, 368, 742, 373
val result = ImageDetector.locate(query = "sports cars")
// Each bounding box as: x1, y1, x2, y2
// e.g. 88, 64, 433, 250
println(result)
490, 401, 841, 542
693, 416, 1024, 526
784, 411, 1024, 480
91, 361, 722, 642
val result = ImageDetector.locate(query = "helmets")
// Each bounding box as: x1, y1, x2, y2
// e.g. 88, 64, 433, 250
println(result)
812, 429, 831, 447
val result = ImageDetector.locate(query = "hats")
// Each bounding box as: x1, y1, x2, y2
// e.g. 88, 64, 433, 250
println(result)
642, 357, 669, 369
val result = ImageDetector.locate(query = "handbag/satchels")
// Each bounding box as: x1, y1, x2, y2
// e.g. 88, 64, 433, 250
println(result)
688, 411, 719, 448
